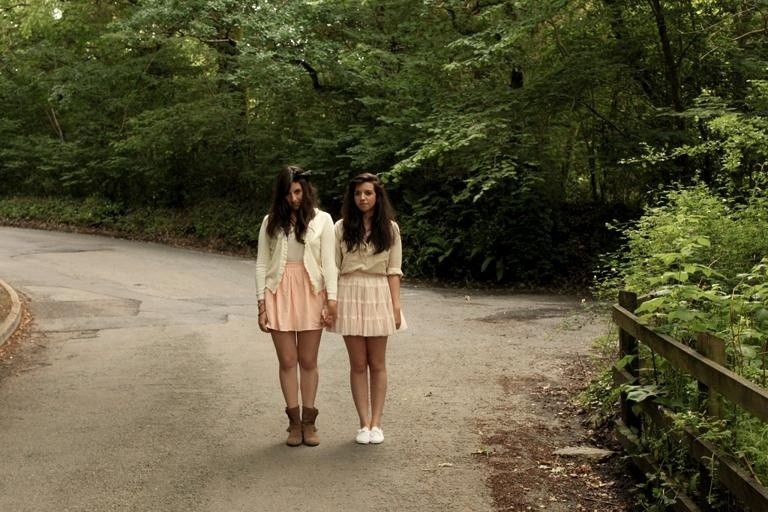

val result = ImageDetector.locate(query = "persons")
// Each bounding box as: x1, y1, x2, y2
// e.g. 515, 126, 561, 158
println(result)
257, 165, 338, 447
319, 172, 403, 446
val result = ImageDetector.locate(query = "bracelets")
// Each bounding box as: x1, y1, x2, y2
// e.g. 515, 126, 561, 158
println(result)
258, 302, 264, 308
258, 310, 266, 315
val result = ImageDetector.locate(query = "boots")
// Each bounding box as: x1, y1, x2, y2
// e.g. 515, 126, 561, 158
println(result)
285, 406, 321, 447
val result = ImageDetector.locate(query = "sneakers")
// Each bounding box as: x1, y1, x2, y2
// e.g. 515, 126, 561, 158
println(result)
355, 425, 385, 445
